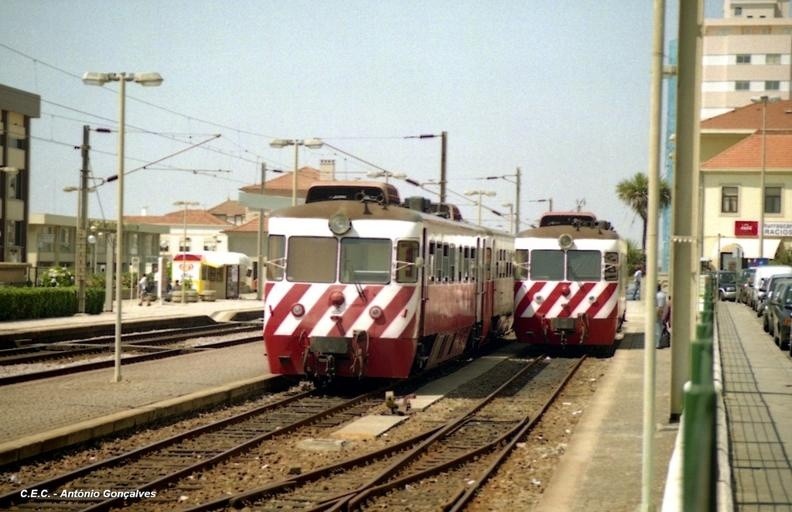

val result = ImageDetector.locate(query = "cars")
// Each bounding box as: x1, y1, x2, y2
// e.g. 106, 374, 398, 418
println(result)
715, 263, 792, 360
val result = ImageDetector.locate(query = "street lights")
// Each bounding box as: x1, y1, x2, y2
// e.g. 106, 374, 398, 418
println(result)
63, 184, 96, 312
751, 91, 782, 258
501, 200, 514, 235
269, 135, 327, 207
173, 198, 201, 303
365, 171, 407, 186
80, 68, 165, 384
463, 187, 497, 224
0, 166, 20, 176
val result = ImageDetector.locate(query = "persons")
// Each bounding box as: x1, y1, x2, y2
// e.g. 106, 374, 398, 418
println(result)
167, 278, 172, 292
174, 279, 179, 289
146, 276, 154, 307
137, 273, 149, 306
632, 266, 642, 301
655, 283, 672, 350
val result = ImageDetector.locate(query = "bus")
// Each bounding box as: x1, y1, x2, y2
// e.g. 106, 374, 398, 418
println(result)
412, 308, 418, 311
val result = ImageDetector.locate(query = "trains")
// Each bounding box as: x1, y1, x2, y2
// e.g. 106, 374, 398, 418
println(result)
509, 211, 626, 359
263, 177, 513, 394
